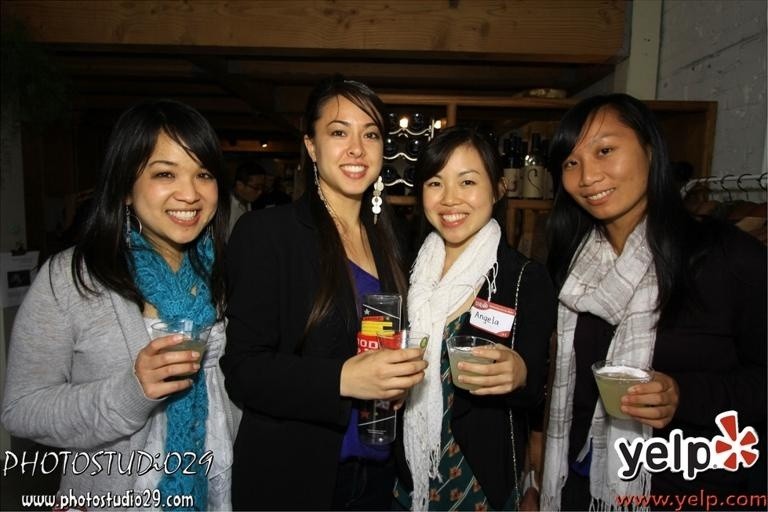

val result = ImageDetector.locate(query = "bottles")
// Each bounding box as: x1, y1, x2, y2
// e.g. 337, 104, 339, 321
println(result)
500, 133, 521, 199
523, 133, 545, 200
357, 295, 402, 444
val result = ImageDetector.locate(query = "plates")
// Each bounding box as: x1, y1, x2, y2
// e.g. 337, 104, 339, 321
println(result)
379, 109, 425, 186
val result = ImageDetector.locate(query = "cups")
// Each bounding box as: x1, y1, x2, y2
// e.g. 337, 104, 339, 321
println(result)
589, 358, 661, 421
377, 328, 430, 363
149, 319, 210, 377
444, 334, 497, 393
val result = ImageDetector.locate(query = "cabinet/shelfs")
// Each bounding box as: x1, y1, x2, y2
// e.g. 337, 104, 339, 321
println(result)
377, 92, 720, 260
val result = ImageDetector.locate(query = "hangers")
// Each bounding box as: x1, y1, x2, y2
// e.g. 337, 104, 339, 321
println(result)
674, 170, 767, 204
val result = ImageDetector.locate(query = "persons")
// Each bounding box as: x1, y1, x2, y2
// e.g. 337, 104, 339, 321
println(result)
220, 161, 264, 245
2, 96, 243, 511
523, 98, 764, 511
279, 165, 306, 200
393, 124, 553, 509
220, 77, 429, 510
